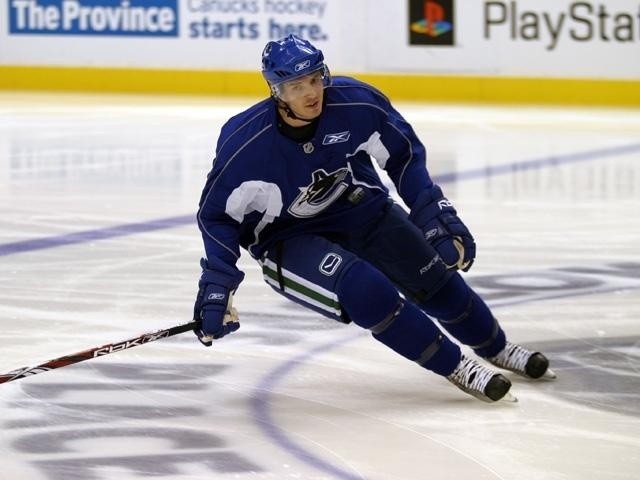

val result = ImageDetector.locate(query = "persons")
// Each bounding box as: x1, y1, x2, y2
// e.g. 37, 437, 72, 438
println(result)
193, 34, 549, 403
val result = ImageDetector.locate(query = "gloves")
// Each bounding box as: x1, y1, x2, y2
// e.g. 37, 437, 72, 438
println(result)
194, 254, 245, 347
407, 184, 476, 272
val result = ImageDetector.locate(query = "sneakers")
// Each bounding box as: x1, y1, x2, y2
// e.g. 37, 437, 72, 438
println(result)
486, 340, 549, 378
447, 354, 511, 403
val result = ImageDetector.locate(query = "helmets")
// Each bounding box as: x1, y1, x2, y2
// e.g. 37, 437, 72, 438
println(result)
261, 34, 333, 103
